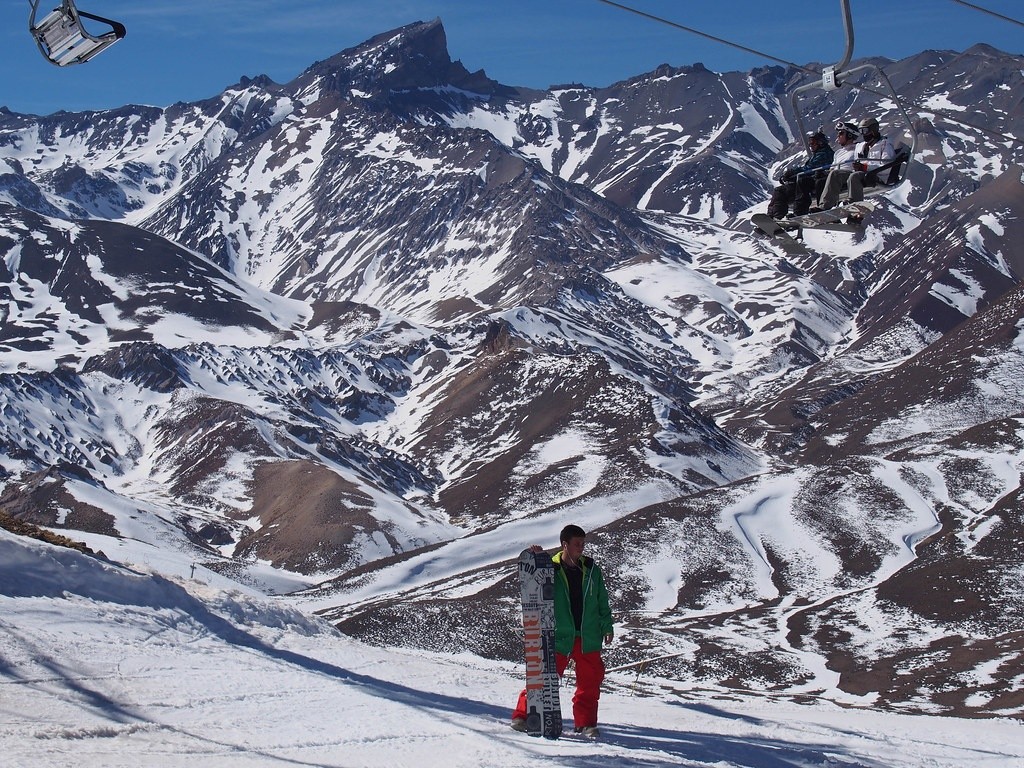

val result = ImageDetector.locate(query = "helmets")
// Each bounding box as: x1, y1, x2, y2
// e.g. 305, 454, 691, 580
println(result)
858, 117, 879, 128
835, 120, 861, 137
805, 130, 827, 140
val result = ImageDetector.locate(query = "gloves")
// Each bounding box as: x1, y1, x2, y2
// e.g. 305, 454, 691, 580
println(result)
851, 162, 864, 171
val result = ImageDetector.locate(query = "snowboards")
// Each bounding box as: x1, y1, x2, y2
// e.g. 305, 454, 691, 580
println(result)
517, 546, 564, 741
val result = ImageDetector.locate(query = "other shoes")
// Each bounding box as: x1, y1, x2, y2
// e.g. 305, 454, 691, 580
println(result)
511, 718, 527, 732
808, 204, 826, 212
573, 726, 600, 739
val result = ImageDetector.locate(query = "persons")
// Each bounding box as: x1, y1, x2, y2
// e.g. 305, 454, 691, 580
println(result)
510, 524, 614, 737
754, 116, 897, 236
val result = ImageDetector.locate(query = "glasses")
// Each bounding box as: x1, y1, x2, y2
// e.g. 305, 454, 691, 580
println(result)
837, 130, 846, 135
